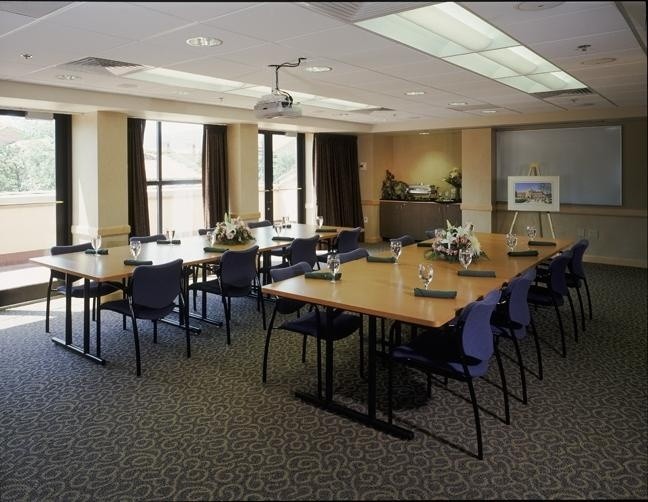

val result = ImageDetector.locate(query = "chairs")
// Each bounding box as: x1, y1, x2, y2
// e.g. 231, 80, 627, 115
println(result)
25, 221, 592, 459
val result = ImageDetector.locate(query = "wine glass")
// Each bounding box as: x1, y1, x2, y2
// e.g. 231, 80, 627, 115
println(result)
418, 262, 436, 291
90, 215, 324, 261
389, 240, 404, 264
434, 226, 537, 271
326, 254, 343, 284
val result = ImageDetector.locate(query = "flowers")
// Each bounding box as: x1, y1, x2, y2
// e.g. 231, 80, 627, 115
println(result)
440, 167, 462, 187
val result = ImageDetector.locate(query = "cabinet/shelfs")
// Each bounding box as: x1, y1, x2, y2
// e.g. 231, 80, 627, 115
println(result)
380, 200, 461, 243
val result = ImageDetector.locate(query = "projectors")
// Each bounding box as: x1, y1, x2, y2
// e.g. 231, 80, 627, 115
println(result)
255, 102, 301, 120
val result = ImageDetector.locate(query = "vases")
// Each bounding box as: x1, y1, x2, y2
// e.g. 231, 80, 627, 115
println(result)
454, 186, 461, 201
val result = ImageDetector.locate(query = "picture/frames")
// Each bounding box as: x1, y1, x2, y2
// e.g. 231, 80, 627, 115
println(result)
505, 172, 562, 215
